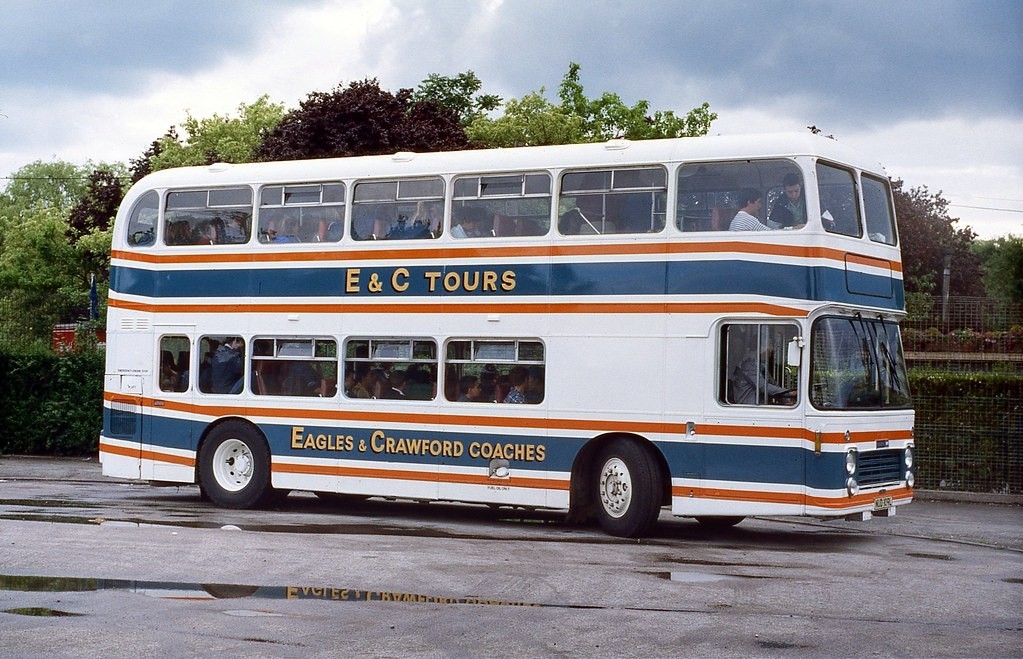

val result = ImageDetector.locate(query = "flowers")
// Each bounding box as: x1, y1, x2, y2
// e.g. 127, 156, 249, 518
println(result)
901, 324, 1023, 346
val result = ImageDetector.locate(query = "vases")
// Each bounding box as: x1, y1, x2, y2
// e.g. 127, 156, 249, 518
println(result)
904, 342, 1023, 352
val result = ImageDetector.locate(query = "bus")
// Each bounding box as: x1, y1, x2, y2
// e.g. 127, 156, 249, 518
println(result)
97, 129, 918, 542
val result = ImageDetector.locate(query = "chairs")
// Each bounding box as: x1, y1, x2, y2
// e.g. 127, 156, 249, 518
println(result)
318, 220, 327, 238
161, 350, 186, 372
516, 217, 538, 235
496, 383, 510, 403
321, 379, 335, 397
256, 361, 272, 395
375, 381, 391, 399
712, 207, 739, 230
493, 214, 515, 237
374, 218, 386, 238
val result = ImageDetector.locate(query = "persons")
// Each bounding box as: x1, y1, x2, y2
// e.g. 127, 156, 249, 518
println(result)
733, 333, 798, 406
729, 186, 807, 232
769, 173, 829, 232
158, 336, 544, 405
189, 207, 488, 247
